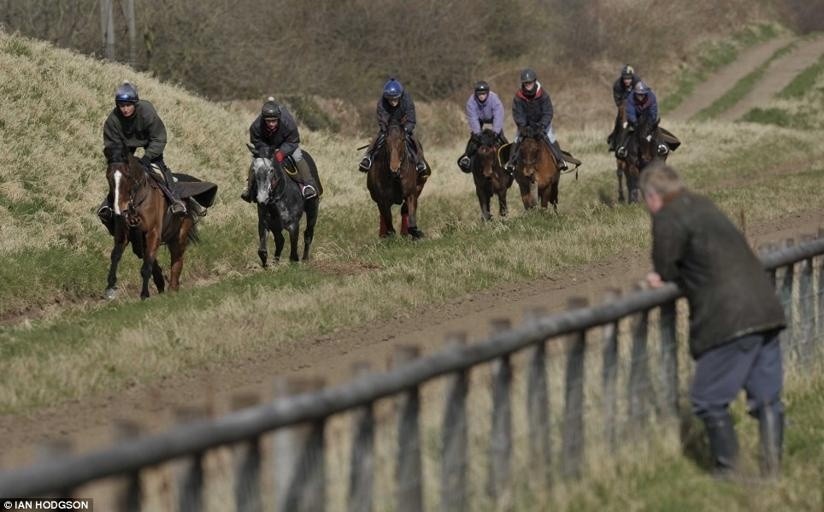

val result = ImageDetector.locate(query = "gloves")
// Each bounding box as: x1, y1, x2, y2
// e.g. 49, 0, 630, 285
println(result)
141, 156, 150, 167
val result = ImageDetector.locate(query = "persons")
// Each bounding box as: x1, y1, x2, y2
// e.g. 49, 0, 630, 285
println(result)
458, 80, 508, 169
616, 80, 670, 158
241, 100, 319, 202
504, 69, 568, 176
359, 78, 429, 177
606, 64, 645, 153
639, 158, 788, 490
98, 83, 189, 221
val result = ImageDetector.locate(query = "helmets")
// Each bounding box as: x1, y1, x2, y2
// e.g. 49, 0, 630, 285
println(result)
475, 80, 489, 94
114, 83, 138, 103
262, 102, 282, 121
622, 64, 634, 79
383, 78, 403, 98
520, 69, 536, 82
634, 81, 649, 94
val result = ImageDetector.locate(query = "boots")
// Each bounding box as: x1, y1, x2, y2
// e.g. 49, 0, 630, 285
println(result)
551, 140, 568, 171
702, 414, 740, 477
164, 168, 185, 217
98, 197, 112, 221
758, 404, 784, 476
297, 159, 319, 197
405, 141, 431, 176
241, 162, 255, 203
505, 141, 521, 172
359, 132, 385, 172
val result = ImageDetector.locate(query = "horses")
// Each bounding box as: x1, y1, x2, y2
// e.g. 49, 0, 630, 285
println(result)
246, 143, 323, 267
472, 129, 514, 222
366, 115, 426, 240
623, 117, 669, 205
513, 126, 560, 216
614, 99, 628, 203
102, 143, 200, 301
